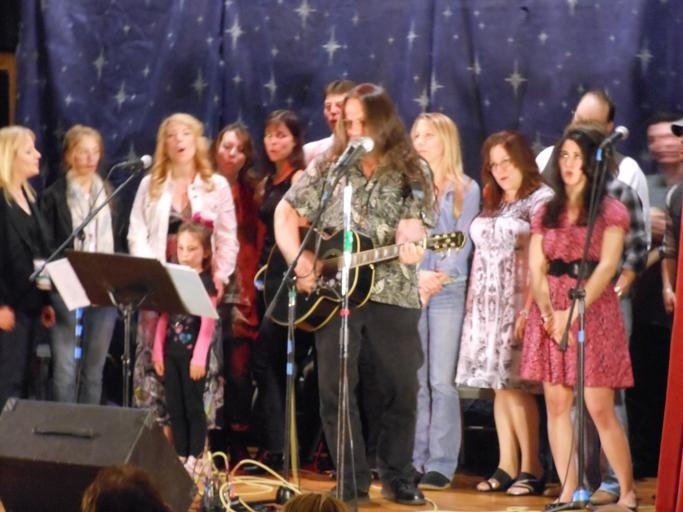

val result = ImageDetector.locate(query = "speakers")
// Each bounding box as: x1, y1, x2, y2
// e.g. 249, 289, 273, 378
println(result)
0, 397, 199, 512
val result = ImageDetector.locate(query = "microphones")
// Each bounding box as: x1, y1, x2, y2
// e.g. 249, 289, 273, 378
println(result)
115, 154, 152, 170
336, 136, 375, 177
331, 140, 361, 175
598, 126, 629, 148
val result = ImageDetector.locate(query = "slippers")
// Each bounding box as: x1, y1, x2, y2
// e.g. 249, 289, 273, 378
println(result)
476, 467, 518, 492
506, 472, 547, 496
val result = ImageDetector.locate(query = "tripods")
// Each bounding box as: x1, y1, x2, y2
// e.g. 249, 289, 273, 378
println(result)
541, 150, 599, 512
199, 178, 348, 512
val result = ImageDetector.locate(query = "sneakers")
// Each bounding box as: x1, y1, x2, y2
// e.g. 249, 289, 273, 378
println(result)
543, 485, 562, 497
417, 471, 452, 490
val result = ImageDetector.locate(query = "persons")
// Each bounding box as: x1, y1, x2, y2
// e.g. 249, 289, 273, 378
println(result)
1, 79, 683, 510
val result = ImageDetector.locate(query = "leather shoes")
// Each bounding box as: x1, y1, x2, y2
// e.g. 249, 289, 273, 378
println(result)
379, 476, 426, 505
330, 482, 369, 506
227, 442, 336, 475
589, 491, 619, 505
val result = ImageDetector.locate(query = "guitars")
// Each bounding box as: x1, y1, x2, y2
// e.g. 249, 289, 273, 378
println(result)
265, 227, 466, 332
